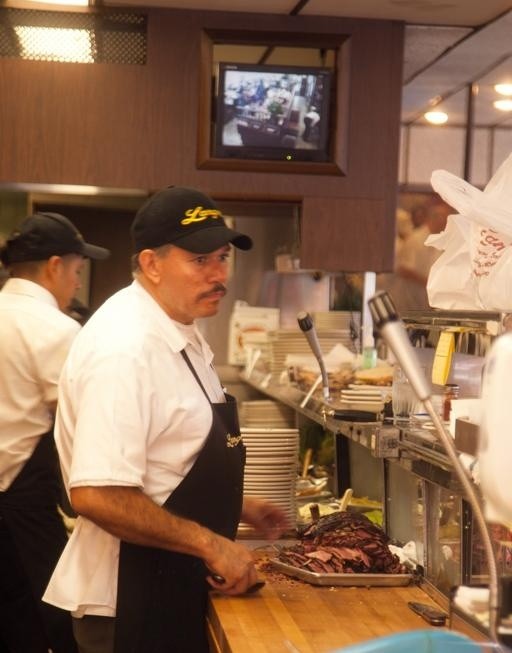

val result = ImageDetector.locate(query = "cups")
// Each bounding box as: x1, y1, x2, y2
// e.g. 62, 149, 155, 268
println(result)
391, 362, 431, 426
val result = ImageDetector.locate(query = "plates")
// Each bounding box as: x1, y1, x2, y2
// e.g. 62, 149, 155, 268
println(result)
270, 325, 359, 386
338, 378, 392, 413
233, 392, 303, 541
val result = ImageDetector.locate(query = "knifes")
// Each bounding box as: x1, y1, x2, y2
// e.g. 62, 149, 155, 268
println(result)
405, 598, 449, 627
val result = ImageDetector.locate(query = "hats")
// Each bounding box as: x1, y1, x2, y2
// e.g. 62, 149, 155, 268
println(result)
5, 212, 111, 263
130, 185, 255, 257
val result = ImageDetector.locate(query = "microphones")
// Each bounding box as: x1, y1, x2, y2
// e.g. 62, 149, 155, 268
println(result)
365, 291, 502, 641
296, 311, 331, 406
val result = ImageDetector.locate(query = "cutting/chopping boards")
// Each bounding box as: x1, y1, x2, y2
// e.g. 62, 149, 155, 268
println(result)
200, 546, 499, 653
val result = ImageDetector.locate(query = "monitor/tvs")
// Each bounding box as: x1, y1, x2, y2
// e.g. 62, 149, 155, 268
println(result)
212, 63, 334, 161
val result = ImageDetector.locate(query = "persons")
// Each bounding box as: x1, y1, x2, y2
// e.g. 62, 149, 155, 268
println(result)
238, 82, 246, 107
2, 210, 111, 653
256, 77, 268, 105
40, 184, 289, 652
394, 201, 456, 284
